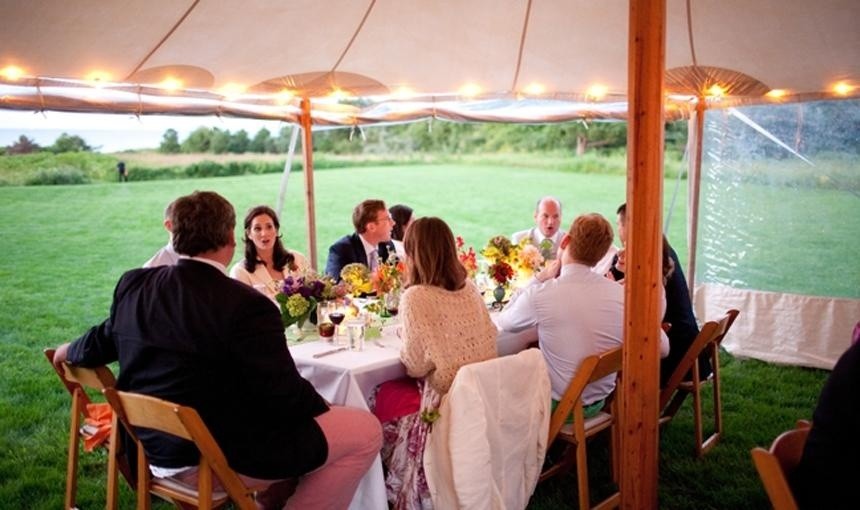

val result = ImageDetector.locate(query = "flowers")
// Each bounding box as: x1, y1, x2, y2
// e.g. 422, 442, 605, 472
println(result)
277, 227, 555, 335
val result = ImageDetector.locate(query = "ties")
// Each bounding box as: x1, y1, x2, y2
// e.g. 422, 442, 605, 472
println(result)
370, 251, 379, 271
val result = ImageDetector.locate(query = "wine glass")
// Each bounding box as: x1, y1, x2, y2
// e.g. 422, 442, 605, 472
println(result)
383, 294, 400, 321
473, 276, 490, 306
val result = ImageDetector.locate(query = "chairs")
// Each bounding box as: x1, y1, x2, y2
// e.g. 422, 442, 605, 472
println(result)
45, 344, 145, 510
538, 324, 672, 509
407, 349, 550, 508
100, 386, 266, 509
661, 309, 741, 456
749, 419, 820, 509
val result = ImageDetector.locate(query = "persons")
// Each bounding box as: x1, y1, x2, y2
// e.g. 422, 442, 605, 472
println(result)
499, 213, 669, 424
389, 204, 416, 259
790, 336, 860, 509
367, 217, 500, 510
512, 197, 565, 260
141, 201, 178, 267
604, 204, 712, 383
228, 205, 312, 315
325, 200, 399, 285
52, 191, 382, 509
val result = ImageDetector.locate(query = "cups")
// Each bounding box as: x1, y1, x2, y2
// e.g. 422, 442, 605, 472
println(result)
318, 299, 364, 351
